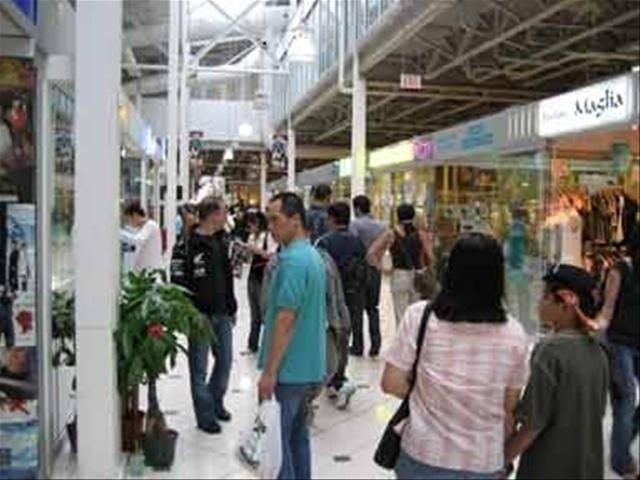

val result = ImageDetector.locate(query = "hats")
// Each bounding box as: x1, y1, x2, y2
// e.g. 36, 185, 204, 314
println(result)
541, 262, 606, 332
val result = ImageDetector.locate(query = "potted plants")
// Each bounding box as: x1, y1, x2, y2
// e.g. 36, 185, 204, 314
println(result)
114, 271, 216, 471
117, 326, 152, 454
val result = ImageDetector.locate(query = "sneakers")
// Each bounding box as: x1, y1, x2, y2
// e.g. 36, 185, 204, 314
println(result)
197, 418, 222, 434
239, 345, 258, 358
216, 405, 231, 424
324, 346, 380, 410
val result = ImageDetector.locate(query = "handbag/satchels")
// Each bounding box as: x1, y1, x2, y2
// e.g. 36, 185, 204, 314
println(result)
236, 392, 285, 480
414, 265, 440, 296
372, 395, 411, 470
378, 247, 393, 272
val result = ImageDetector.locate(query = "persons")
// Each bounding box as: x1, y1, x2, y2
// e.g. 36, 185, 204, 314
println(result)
122, 182, 437, 480
504, 263, 609, 480
595, 225, 640, 476
380, 232, 527, 480
